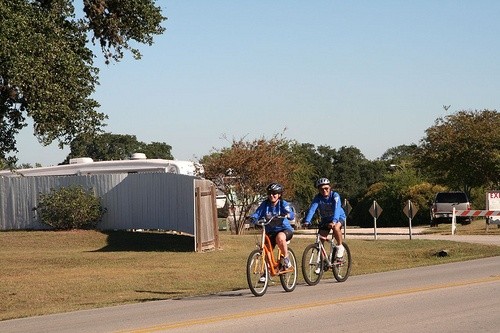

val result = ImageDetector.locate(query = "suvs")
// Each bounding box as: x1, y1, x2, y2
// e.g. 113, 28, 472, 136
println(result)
427, 192, 471, 226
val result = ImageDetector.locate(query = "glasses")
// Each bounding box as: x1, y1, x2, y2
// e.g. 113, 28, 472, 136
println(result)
267, 192, 278, 195
320, 187, 329, 191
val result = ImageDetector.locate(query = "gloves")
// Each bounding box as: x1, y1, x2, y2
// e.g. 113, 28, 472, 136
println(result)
248, 216, 259, 225
280, 212, 289, 218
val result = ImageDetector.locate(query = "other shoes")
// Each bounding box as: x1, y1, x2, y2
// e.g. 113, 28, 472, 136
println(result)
336, 245, 346, 258
282, 256, 293, 269
314, 267, 321, 274
259, 271, 267, 283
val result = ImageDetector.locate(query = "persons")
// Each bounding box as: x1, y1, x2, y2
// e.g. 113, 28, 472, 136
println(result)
245, 182, 296, 282
301, 176, 347, 274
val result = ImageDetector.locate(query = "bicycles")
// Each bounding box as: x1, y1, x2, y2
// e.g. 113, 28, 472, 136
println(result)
301, 224, 352, 287
246, 215, 298, 297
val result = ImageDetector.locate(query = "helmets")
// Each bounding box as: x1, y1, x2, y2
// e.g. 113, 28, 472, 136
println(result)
315, 177, 330, 187
265, 182, 285, 193
